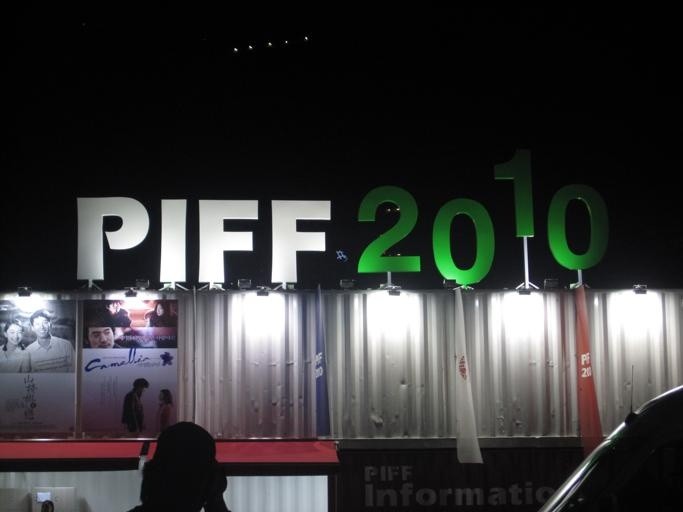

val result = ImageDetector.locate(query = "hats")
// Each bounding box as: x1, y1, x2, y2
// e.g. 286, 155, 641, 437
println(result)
134, 378, 149, 387
145, 422, 227, 495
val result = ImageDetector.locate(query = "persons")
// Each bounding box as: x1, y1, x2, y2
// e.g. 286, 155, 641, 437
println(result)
153, 387, 174, 437
120, 376, 149, 433
16, 306, 76, 374
83, 300, 132, 327
126, 419, 235, 512
83, 325, 122, 349
143, 301, 176, 329
0, 318, 32, 373
40, 499, 54, 511
